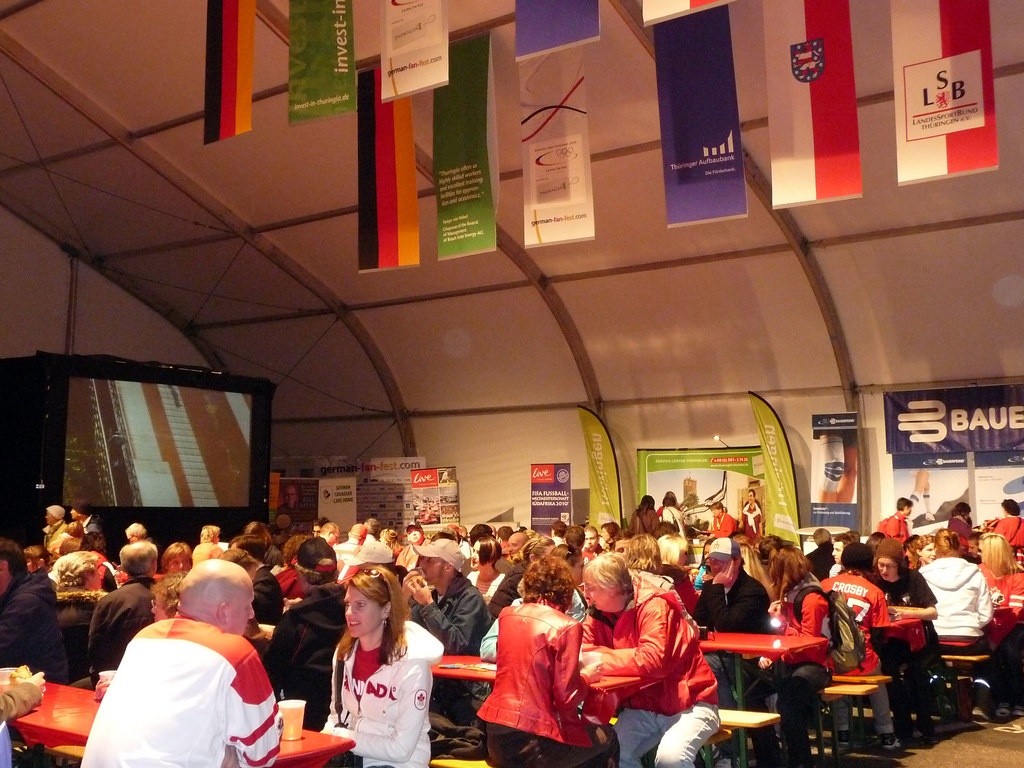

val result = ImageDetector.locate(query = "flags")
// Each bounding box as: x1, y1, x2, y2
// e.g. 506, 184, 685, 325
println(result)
576, 404, 621, 535
356, 67, 419, 273
433, 34, 496, 263
889, 1, 999, 188
747, 391, 802, 547
514, 0, 601, 61
654, 2, 748, 230
204, 1, 255, 145
377, 1, 449, 105
287, 0, 358, 126
760, 1, 862, 210
514, 45, 596, 249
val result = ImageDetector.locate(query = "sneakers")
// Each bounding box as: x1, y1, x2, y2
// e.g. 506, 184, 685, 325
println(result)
880, 734, 900, 750
996, 704, 1024, 716
972, 708, 990, 720
834, 731, 851, 752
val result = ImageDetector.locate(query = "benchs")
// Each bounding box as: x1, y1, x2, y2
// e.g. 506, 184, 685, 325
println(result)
832, 675, 892, 752
718, 707, 781, 729
941, 655, 989, 717
608, 717, 731, 752
815, 683, 880, 768
430, 759, 491, 768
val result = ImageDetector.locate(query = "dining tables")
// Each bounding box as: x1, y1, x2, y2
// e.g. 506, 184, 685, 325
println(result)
432, 656, 641, 696
9, 681, 357, 768
857, 619, 922, 747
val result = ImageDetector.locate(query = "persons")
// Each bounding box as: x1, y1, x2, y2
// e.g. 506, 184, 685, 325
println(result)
1, 489, 1023, 768
906, 469, 937, 527
277, 484, 302, 522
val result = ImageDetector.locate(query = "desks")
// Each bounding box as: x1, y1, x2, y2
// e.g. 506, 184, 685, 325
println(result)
699, 632, 829, 768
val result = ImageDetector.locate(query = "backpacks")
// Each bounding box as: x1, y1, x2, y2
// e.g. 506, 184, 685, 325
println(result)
794, 586, 866, 674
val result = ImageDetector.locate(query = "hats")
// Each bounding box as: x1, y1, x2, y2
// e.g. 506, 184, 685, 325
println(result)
876, 536, 903, 562
412, 539, 465, 572
406, 519, 423, 532
72, 500, 92, 515
291, 536, 337, 570
704, 537, 741, 562
46, 505, 66, 519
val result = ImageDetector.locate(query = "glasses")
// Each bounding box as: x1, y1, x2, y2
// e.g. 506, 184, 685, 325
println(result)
356, 568, 386, 584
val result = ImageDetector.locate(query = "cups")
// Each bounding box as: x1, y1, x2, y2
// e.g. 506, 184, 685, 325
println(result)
98, 670, 118, 684
693, 539, 699, 545
278, 699, 307, 741
0, 667, 20, 685
